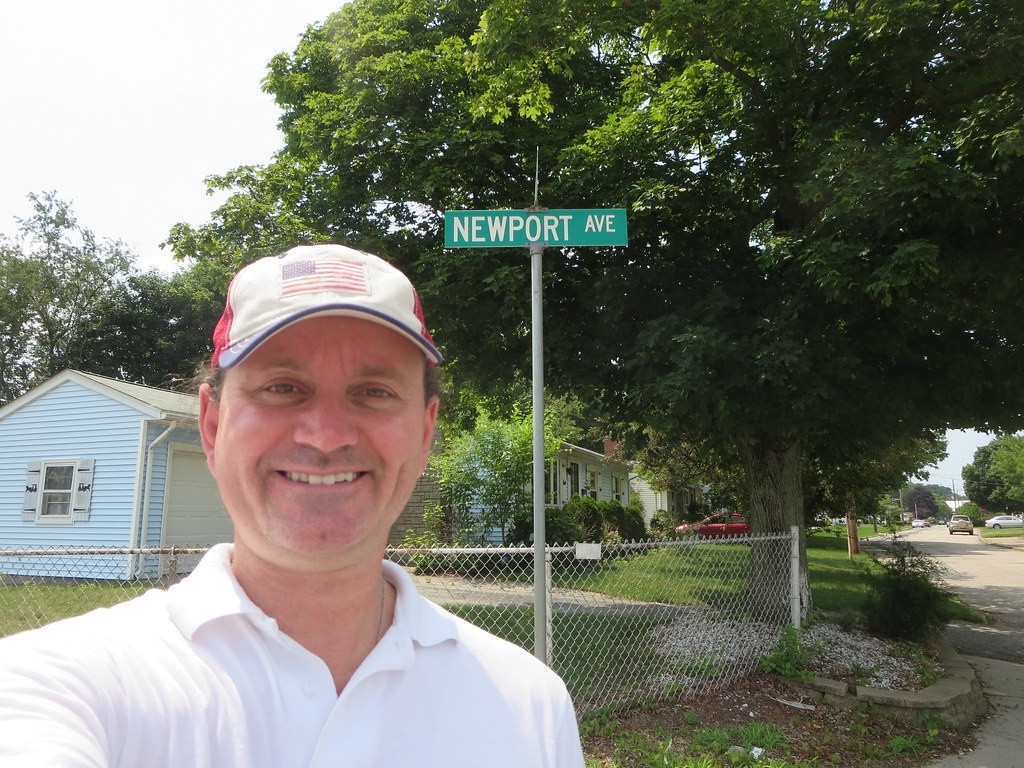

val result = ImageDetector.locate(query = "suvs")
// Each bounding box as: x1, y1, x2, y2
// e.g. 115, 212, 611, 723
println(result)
949, 515, 974, 535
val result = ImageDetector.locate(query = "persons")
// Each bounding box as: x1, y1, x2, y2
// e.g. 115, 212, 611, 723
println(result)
1, 245, 586, 768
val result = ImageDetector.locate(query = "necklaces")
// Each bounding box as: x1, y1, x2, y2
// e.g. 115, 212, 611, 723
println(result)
377, 580, 386, 642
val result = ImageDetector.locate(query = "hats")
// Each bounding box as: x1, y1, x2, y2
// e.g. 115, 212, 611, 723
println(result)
211, 243, 443, 371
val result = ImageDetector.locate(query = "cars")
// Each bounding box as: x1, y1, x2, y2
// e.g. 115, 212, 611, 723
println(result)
985, 516, 1024, 529
923, 519, 930, 527
675, 512, 751, 539
912, 520, 924, 528
